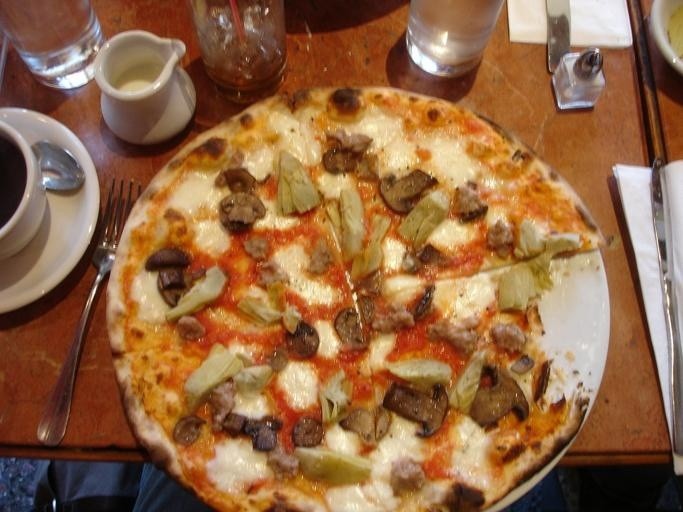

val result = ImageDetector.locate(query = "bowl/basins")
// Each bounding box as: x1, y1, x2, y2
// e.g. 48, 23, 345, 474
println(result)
649, 0, 683, 76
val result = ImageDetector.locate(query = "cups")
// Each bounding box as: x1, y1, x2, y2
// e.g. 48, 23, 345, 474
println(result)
191, 0, 287, 104
0, 0, 103, 90
0, 118, 46, 262
95, 30, 198, 147
406, 0, 505, 78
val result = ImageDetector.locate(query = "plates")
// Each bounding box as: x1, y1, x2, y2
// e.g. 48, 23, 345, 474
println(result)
0, 106, 101, 314
482, 246, 616, 512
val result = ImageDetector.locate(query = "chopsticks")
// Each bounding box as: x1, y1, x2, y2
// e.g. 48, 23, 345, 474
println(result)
627, 0, 669, 165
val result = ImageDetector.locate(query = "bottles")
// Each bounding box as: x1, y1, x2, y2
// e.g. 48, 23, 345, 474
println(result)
550, 45, 607, 111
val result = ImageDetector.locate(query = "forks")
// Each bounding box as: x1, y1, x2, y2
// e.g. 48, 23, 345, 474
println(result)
37, 176, 143, 444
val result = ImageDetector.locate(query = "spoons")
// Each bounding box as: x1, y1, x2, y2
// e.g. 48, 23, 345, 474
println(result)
29, 141, 85, 192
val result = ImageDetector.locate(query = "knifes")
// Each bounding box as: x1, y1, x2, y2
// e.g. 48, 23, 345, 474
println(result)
545, 0, 571, 73
649, 154, 683, 455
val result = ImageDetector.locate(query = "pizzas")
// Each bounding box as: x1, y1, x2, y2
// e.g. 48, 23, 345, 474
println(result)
105, 87, 607, 512
